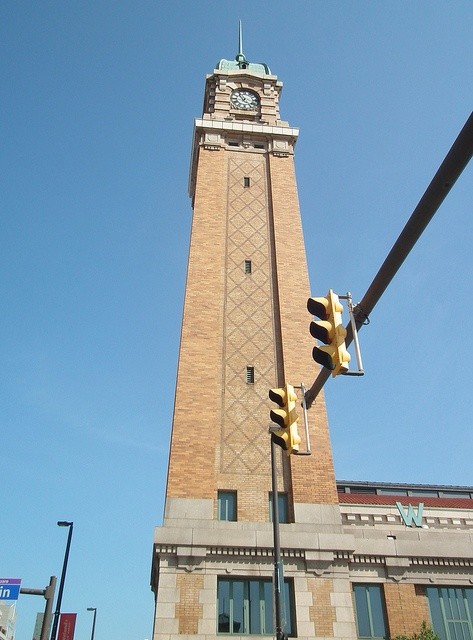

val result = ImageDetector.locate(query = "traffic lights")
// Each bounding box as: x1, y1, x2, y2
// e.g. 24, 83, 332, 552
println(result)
268, 383, 301, 458
307, 288, 350, 379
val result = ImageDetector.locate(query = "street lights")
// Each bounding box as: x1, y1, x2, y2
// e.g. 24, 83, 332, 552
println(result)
86, 608, 96, 640
49, 521, 73, 640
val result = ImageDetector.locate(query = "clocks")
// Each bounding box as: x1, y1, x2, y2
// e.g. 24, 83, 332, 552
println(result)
230, 86, 259, 114
